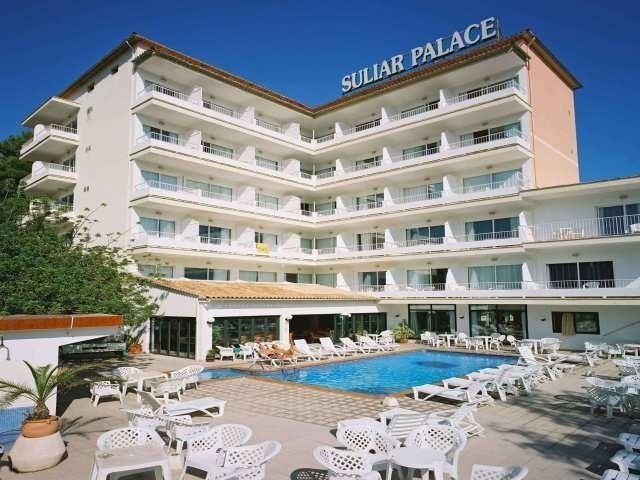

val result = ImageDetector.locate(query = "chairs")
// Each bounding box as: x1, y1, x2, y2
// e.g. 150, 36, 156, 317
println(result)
181, 424, 252, 477
380, 410, 430, 437
204, 440, 282, 480
166, 415, 209, 454
122, 406, 161, 428
471, 461, 524, 479
112, 365, 140, 382
572, 337, 640, 479
405, 425, 467, 474
336, 419, 403, 479
213, 326, 575, 414
97, 426, 164, 452
311, 443, 380, 480
171, 364, 203, 389
84, 377, 124, 406
435, 403, 484, 436
134, 387, 225, 417
150, 378, 185, 401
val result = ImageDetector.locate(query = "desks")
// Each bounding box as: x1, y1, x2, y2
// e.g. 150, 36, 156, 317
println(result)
125, 369, 167, 401
386, 447, 447, 480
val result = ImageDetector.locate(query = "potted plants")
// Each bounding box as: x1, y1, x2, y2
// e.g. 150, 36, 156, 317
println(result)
0, 357, 130, 441
